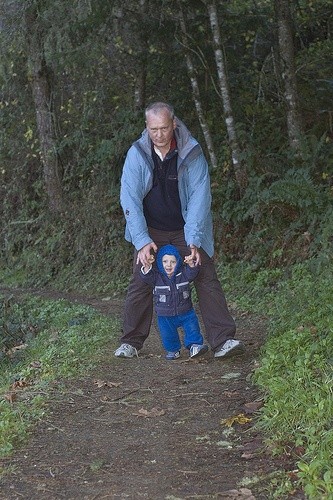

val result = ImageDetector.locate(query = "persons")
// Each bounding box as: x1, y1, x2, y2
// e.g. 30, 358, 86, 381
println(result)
113, 101, 245, 360
139, 244, 210, 360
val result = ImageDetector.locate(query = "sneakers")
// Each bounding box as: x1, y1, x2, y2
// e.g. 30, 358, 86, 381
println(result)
214, 339, 245, 358
112, 343, 138, 358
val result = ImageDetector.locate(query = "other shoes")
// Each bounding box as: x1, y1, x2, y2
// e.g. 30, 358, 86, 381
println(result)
189, 345, 208, 358
165, 350, 180, 360
